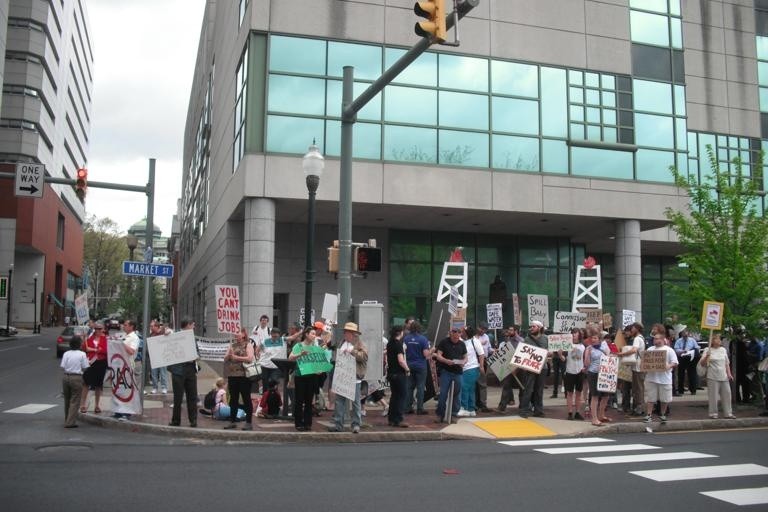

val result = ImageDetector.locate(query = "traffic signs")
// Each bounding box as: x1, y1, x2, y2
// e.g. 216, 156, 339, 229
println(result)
14, 163, 44, 198
123, 261, 174, 279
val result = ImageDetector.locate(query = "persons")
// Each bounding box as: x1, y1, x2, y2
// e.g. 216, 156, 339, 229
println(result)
60, 315, 144, 428
549, 314, 702, 426
200, 315, 368, 432
700, 324, 767, 419
361, 318, 492, 428
150, 317, 198, 427
495, 321, 549, 416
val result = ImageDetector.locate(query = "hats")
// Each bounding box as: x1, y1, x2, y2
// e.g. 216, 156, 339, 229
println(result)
314, 322, 323, 329
341, 322, 361, 336
461, 326, 473, 338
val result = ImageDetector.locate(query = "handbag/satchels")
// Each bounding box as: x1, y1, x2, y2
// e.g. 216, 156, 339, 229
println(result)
242, 362, 262, 382
696, 357, 706, 377
479, 356, 487, 373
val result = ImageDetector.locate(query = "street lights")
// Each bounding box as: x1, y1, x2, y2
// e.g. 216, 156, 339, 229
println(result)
126, 236, 138, 319
32, 273, 39, 334
96, 269, 108, 312
302, 137, 325, 343
6, 263, 13, 334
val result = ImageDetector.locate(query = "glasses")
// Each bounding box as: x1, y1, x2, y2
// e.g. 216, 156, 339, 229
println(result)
95, 328, 102, 330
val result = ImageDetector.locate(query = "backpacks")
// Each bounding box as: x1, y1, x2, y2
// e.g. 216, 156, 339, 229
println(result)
204, 388, 222, 410
267, 391, 279, 415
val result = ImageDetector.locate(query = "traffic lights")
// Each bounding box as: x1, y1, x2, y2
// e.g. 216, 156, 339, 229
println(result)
74, 168, 87, 199
353, 246, 382, 274
412, 0, 436, 37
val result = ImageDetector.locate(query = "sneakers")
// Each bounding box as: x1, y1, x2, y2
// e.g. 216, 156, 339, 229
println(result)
242, 423, 252, 430
162, 389, 167, 394
496, 400, 544, 418
479, 405, 493, 412
432, 408, 476, 424
224, 423, 236, 429
383, 404, 428, 428
192, 423, 196, 426
709, 415, 720, 419
724, 415, 736, 419
328, 401, 366, 433
673, 386, 704, 396
550, 394, 557, 398
81, 407, 87, 413
108, 413, 129, 420
568, 402, 671, 426
150, 389, 157, 394
168, 421, 179, 426
95, 408, 101, 413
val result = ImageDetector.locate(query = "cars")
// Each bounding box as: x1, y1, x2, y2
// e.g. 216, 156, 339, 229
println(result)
0, 325, 18, 336
57, 326, 92, 356
94, 317, 120, 335
